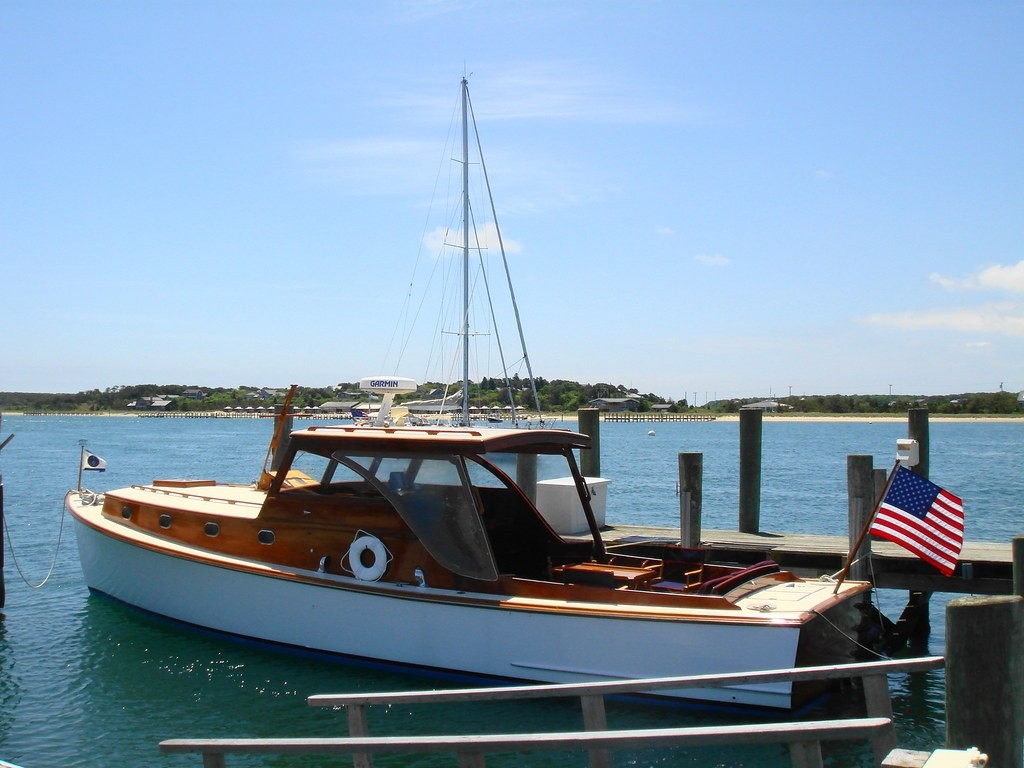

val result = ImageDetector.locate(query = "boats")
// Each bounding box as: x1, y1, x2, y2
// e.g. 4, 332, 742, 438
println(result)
64, 386, 874, 713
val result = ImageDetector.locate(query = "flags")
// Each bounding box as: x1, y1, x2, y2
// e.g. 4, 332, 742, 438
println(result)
81, 448, 108, 472
868, 464, 964, 577
